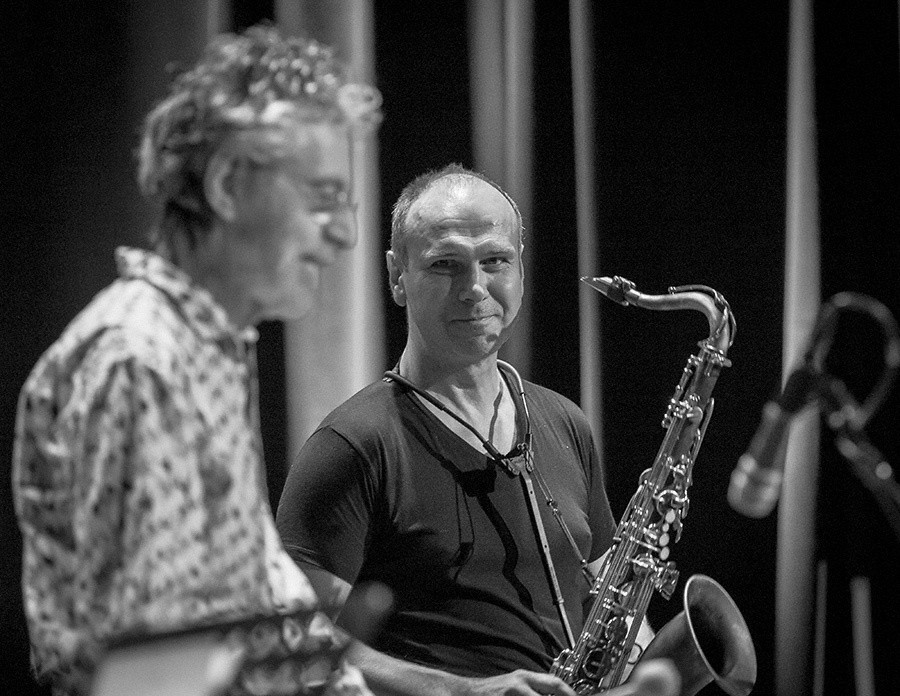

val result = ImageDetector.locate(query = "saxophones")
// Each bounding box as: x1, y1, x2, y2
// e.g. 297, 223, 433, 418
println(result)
550, 275, 757, 696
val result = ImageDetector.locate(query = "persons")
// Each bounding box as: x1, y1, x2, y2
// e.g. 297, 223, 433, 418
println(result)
275, 161, 680, 696
12, 24, 383, 696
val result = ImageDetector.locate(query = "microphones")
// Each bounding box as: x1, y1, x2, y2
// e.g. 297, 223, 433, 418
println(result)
723, 307, 838, 518
